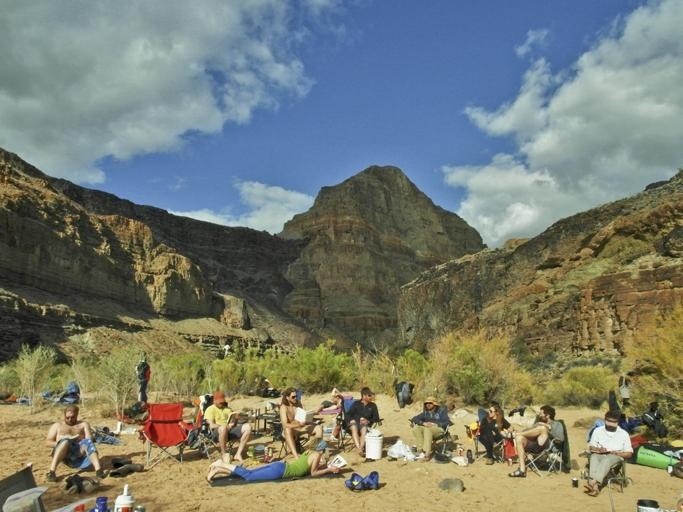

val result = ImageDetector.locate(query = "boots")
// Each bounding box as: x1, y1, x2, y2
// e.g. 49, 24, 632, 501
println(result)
110, 463, 144, 477
111, 457, 133, 468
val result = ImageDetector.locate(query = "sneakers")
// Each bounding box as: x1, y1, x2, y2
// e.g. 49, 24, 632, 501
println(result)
500, 431, 515, 439
509, 467, 527, 477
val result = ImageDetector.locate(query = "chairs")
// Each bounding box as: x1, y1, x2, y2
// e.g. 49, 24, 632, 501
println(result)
193, 400, 235, 460
269, 403, 308, 457
140, 403, 191, 469
318, 395, 384, 452
429, 418, 630, 493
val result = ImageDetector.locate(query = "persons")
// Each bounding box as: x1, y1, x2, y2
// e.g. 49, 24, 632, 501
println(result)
204, 438, 339, 481
44, 405, 109, 482
280, 387, 381, 459
479, 404, 564, 477
411, 396, 449, 460
584, 410, 633, 495
203, 390, 251, 463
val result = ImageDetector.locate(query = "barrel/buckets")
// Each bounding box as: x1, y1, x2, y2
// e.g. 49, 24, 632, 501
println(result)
365, 434, 384, 460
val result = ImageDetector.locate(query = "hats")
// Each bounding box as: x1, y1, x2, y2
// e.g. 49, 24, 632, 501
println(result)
214, 391, 226, 404
424, 397, 439, 406
605, 413, 620, 428
361, 387, 374, 394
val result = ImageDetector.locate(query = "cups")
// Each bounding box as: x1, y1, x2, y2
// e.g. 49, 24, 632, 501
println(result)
571, 479, 579, 488
508, 458, 512, 466
410, 446, 416, 453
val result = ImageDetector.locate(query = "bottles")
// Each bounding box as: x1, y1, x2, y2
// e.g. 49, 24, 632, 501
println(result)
359, 418, 364, 425
89, 496, 109, 512
268, 448, 272, 456
467, 449, 472, 464
264, 447, 268, 455
112, 483, 136, 511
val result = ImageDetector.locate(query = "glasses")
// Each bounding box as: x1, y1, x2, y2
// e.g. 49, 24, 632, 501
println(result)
292, 395, 297, 399
